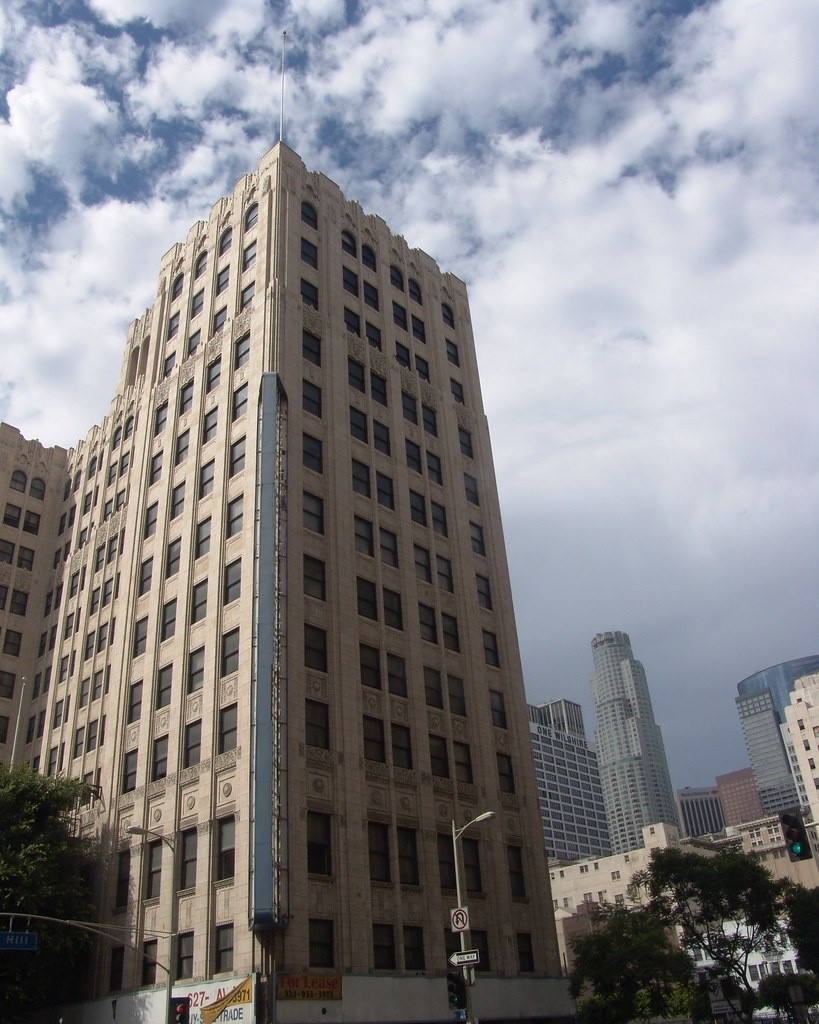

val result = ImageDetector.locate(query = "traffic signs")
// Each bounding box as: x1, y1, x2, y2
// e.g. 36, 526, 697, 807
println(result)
449, 948, 481, 967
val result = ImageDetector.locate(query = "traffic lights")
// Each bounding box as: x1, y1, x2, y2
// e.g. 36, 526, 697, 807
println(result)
775, 805, 814, 863
446, 963, 467, 1011
169, 996, 191, 1024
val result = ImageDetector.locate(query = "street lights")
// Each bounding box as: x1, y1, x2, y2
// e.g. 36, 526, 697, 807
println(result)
124, 827, 177, 1024
451, 810, 496, 1024
